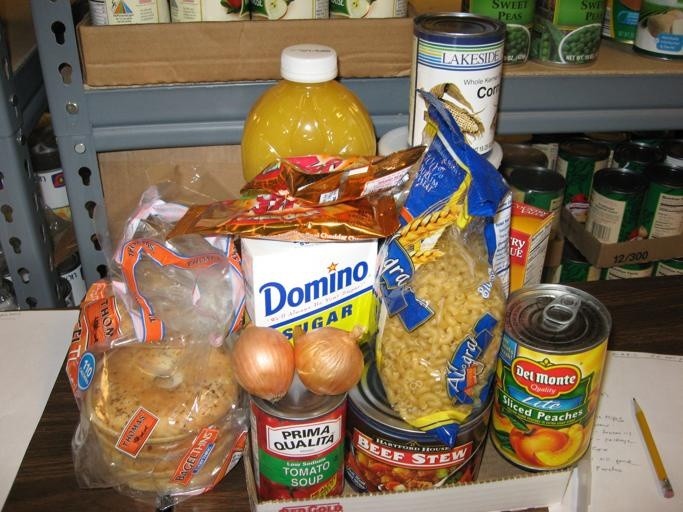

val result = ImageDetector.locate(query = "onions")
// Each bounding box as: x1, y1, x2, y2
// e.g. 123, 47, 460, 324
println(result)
232, 326, 295, 404
293, 327, 365, 396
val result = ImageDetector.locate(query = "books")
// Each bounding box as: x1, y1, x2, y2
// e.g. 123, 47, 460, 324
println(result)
242, 457, 572, 508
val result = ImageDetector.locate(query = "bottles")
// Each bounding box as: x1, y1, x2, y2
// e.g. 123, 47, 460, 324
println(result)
583, 168, 645, 245
531, 0, 607, 68
602, 1, 646, 45
508, 166, 566, 242
489, 284, 612, 473
633, 0, 683, 60
557, 136, 614, 224
604, 262, 655, 280
408, 11, 506, 159
88, 1, 170, 27
248, 384, 347, 502
242, 44, 376, 185
166, 0, 251, 22
636, 165, 681, 239
657, 259, 683, 277
610, 140, 664, 171
666, 137, 683, 167
347, 354, 495, 494
248, 0, 332, 22
534, 134, 561, 170
560, 254, 599, 283
329, 0, 409, 22
460, 0, 532, 67
500, 143, 549, 177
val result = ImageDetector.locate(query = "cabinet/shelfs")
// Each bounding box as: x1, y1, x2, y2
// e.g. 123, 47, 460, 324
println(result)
31, 0, 681, 289
0, 0, 78, 310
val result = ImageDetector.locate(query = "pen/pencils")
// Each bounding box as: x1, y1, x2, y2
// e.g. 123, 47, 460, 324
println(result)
633, 397, 674, 499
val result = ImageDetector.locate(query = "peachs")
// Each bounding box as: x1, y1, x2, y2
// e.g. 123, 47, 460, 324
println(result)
502, 409, 568, 466
509, 424, 545, 452
534, 424, 584, 467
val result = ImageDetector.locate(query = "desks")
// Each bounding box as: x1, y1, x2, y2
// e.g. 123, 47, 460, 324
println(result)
2, 278, 680, 512
